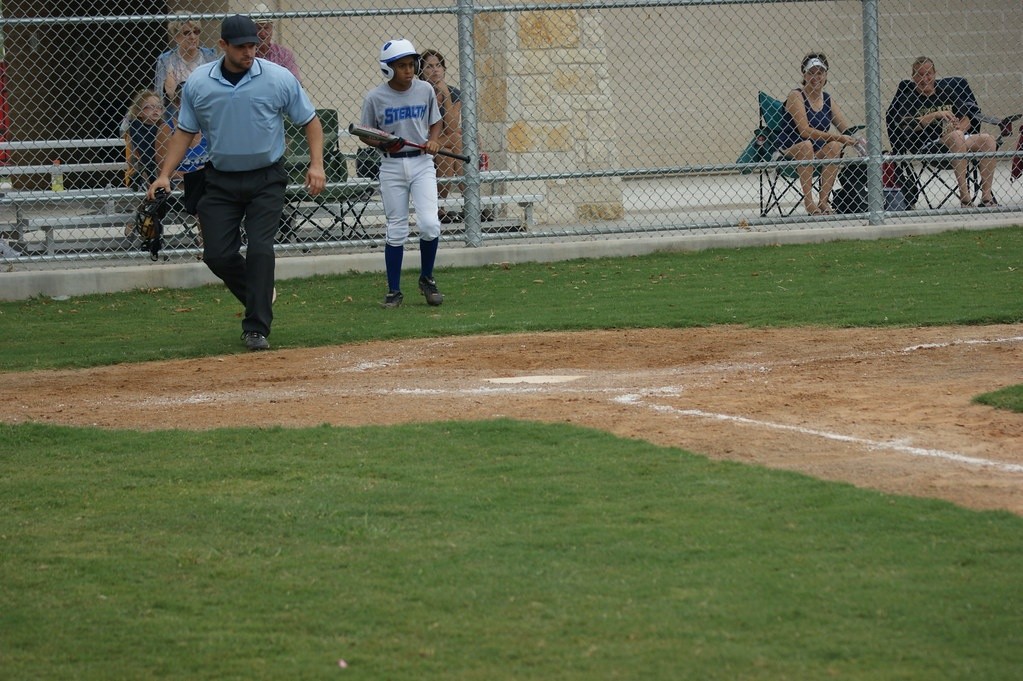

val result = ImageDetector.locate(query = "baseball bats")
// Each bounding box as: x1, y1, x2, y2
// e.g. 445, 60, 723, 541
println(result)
347, 122, 472, 164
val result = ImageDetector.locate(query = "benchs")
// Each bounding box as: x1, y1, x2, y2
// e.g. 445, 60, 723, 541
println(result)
0, 138, 547, 255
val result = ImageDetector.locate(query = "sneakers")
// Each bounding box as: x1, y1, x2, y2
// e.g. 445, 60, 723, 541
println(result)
419, 276, 443, 307
381, 292, 405, 311
242, 329, 269, 351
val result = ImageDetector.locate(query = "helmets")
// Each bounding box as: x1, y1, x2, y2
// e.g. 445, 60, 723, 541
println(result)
380, 39, 421, 81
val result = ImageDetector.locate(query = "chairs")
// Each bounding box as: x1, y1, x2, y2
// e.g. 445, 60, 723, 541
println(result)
889, 79, 1023, 209
276, 108, 377, 251
737, 89, 866, 218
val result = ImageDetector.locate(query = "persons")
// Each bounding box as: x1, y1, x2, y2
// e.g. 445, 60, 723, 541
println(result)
772, 52, 865, 217
897, 56, 1002, 209
145, 14, 327, 352
418, 50, 465, 221
120, 4, 304, 251
359, 40, 443, 308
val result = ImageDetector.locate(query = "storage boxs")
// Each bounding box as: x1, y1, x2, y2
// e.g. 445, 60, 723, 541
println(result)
881, 188, 906, 210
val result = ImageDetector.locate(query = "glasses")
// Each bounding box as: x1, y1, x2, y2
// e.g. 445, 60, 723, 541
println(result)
179, 28, 202, 37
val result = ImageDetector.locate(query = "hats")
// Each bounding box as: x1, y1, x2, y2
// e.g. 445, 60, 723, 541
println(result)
802, 57, 829, 71
249, 2, 274, 23
221, 14, 259, 48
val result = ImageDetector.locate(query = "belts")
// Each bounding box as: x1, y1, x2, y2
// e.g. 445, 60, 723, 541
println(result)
383, 150, 425, 159
